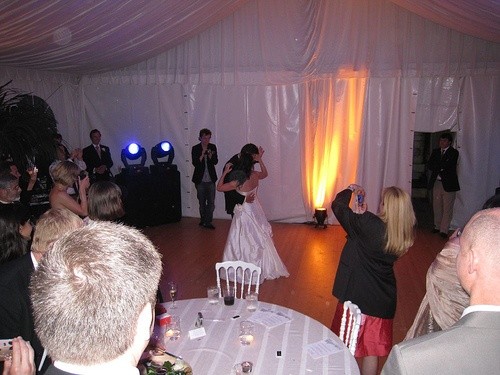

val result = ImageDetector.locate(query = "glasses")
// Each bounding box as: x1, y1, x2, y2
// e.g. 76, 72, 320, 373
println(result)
2, 185, 19, 191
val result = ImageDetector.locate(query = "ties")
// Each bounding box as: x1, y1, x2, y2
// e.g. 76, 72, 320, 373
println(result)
442, 150, 445, 155
96, 145, 102, 159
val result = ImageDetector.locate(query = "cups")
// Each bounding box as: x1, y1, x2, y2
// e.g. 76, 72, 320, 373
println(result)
240, 321, 255, 346
234, 361, 254, 375
207, 286, 219, 304
165, 316, 181, 341
223, 285, 235, 306
246, 291, 257, 312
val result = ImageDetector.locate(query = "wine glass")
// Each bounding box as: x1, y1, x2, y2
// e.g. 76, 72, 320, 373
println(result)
168, 284, 177, 309
38, 176, 47, 196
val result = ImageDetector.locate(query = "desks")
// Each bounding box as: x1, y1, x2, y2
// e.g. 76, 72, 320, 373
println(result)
140, 291, 362, 375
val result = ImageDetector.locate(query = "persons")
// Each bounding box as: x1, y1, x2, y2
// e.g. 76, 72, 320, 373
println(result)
217, 142, 290, 285
0, 133, 126, 375
27, 216, 163, 375
426, 132, 460, 238
82, 128, 114, 188
378, 194, 500, 375
330, 183, 416, 375
191, 128, 219, 230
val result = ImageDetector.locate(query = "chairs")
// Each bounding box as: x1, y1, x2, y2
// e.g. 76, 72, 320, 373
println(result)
338, 301, 359, 356
215, 261, 262, 301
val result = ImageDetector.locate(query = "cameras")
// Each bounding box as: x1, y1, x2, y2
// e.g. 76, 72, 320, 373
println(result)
0, 339, 14, 361
356, 194, 364, 207
79, 170, 87, 180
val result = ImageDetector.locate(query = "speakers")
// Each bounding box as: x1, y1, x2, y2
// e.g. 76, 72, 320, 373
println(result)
411, 130, 455, 229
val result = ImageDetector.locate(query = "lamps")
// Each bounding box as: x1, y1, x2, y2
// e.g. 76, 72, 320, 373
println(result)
120, 143, 144, 170
152, 141, 175, 169
313, 207, 329, 227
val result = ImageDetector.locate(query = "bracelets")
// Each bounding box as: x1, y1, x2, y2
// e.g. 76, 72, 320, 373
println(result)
348, 185, 356, 192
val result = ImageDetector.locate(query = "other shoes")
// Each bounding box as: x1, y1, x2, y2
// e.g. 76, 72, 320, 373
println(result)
431, 229, 440, 233
440, 232, 448, 238
205, 224, 216, 229
200, 220, 204, 225
